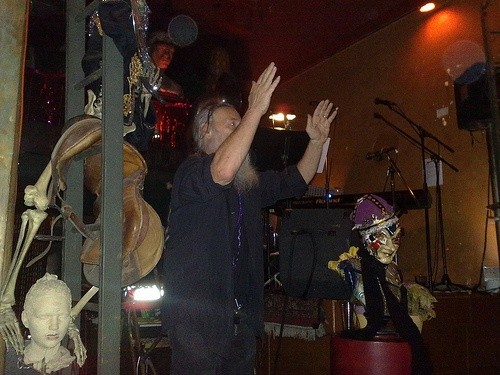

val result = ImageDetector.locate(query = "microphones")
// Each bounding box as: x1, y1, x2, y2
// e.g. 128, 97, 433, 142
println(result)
366, 149, 396, 160
374, 97, 397, 107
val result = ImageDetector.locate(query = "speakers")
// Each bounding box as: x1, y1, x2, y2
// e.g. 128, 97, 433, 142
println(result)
278, 208, 363, 301
454, 62, 492, 131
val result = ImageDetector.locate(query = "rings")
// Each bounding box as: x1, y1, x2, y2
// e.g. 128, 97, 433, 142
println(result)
323, 115, 328, 118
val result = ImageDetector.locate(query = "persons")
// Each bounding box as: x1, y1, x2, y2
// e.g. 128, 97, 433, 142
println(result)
21, 272, 76, 374
328, 193, 408, 327
161, 61, 337, 375
146, 31, 184, 98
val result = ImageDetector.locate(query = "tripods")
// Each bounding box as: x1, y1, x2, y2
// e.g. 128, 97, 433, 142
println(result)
373, 112, 472, 293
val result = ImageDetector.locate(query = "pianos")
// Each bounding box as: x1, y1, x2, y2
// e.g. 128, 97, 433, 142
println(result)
272, 190, 434, 214
